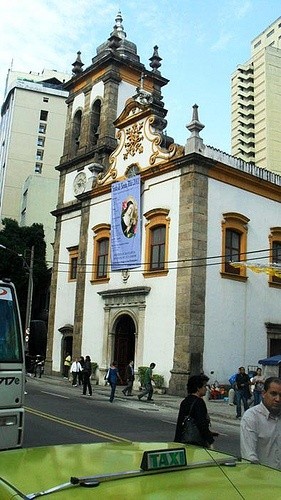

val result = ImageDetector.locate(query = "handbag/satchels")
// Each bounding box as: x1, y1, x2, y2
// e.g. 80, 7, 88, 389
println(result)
179, 397, 213, 444
106, 368, 110, 380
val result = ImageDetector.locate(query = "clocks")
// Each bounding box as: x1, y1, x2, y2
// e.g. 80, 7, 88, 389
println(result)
72, 172, 88, 197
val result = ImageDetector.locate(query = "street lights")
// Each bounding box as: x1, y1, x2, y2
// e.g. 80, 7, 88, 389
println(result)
0, 244, 33, 360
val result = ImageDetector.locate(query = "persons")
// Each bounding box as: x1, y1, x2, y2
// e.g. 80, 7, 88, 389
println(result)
138, 363, 157, 402
174, 373, 222, 453
62, 352, 71, 380
34, 354, 44, 378
240, 377, 281, 469
122, 360, 135, 396
25, 350, 31, 374
234, 367, 266, 418
69, 356, 92, 396
106, 362, 120, 402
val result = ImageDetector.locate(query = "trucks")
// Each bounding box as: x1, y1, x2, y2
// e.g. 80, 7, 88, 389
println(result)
0, 278, 32, 449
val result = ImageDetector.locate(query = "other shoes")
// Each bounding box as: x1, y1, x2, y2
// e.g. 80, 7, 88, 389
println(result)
80, 393, 83, 395
236, 414, 241, 418
127, 394, 133, 396
122, 390, 126, 396
138, 394, 140, 400
146, 398, 153, 401
64, 377, 68, 380
87, 394, 90, 396
73, 384, 77, 387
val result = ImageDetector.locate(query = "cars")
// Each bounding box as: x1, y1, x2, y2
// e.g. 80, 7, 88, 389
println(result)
0, 441, 281, 500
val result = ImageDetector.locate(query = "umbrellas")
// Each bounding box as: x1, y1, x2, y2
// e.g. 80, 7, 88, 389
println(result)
259, 355, 281, 366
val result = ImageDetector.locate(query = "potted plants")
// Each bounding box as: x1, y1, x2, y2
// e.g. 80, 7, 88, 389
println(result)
152, 374, 166, 395
138, 367, 149, 391
89, 362, 98, 385
